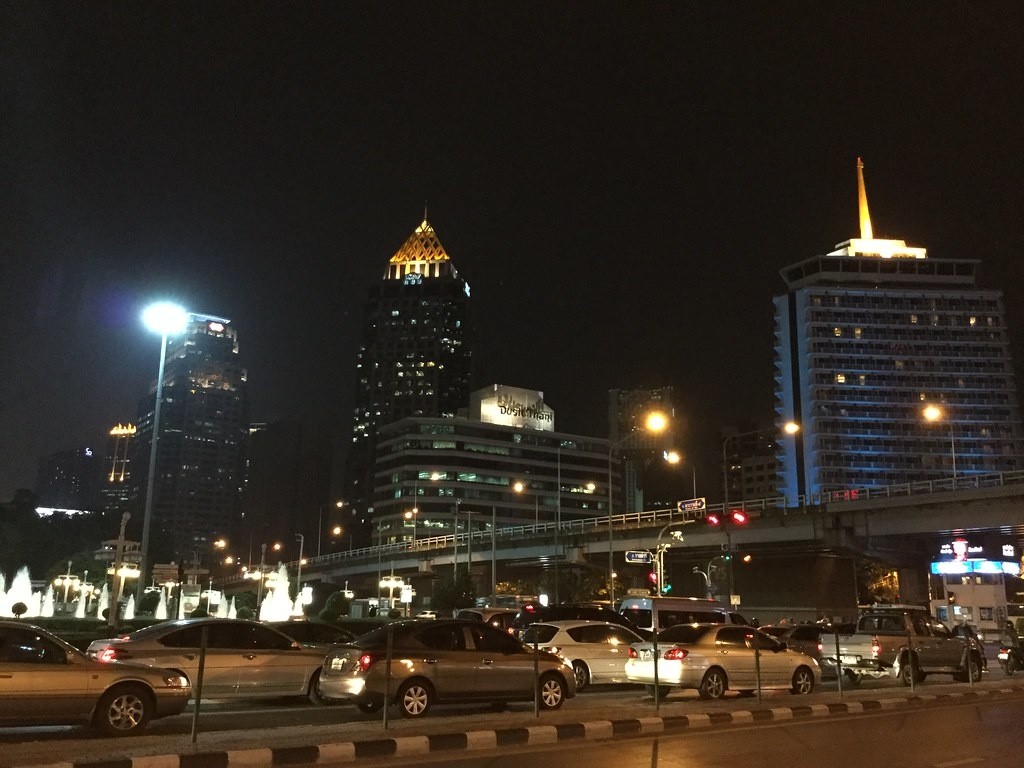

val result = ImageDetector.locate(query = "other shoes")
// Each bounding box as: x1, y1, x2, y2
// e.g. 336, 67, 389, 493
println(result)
982, 668, 990, 673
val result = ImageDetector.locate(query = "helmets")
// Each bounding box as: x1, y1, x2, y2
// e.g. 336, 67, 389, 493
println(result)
959, 615, 968, 625
750, 617, 760, 627
1005, 620, 1014, 629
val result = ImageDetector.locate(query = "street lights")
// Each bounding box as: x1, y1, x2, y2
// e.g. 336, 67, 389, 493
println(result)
608, 413, 666, 612
722, 423, 799, 604
413, 473, 439, 547
923, 405, 956, 488
317, 501, 343, 559
133, 304, 190, 615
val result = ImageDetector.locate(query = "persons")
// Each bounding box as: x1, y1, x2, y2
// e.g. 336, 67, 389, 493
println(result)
751, 617, 761, 629
368, 604, 379, 617
998, 620, 1024, 668
788, 615, 833, 625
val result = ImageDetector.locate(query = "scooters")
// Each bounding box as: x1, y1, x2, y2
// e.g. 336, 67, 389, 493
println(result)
997, 637, 1024, 676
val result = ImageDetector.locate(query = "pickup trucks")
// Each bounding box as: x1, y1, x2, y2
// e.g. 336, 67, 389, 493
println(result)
816, 603, 983, 686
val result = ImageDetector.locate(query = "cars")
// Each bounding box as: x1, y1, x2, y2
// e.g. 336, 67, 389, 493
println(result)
754, 623, 832, 658
504, 603, 645, 644
624, 622, 822, 700
87, 616, 338, 708
0, 620, 192, 740
454, 607, 521, 633
270, 621, 359, 651
319, 618, 579, 720
519, 619, 647, 693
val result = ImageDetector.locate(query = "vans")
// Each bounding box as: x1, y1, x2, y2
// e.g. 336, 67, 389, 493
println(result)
618, 595, 757, 635
